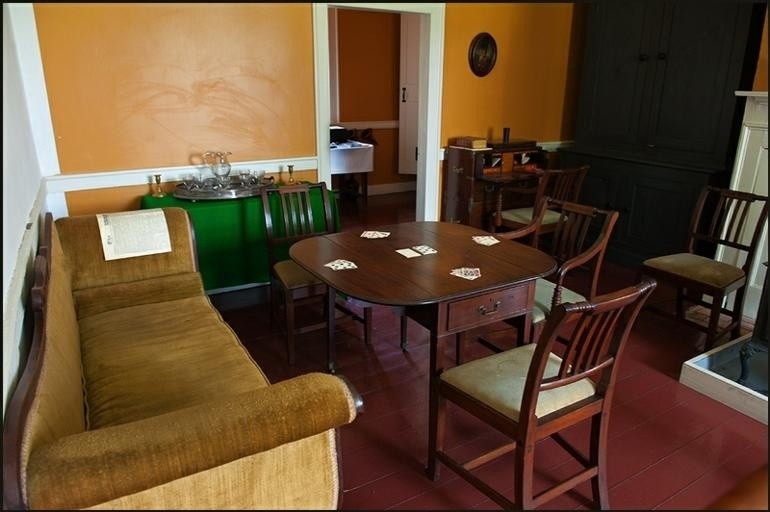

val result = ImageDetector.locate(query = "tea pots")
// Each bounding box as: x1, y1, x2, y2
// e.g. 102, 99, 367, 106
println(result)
203, 150, 233, 191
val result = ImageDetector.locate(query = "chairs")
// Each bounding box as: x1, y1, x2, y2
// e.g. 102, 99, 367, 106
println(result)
258, 181, 374, 363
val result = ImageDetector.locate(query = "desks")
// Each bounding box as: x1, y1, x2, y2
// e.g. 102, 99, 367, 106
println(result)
290, 221, 560, 484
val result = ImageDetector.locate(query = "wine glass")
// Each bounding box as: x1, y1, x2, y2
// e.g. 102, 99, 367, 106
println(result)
181, 173, 202, 194
238, 168, 265, 190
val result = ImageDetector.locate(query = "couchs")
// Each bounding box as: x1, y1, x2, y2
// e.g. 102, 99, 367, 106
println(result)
2, 209, 367, 510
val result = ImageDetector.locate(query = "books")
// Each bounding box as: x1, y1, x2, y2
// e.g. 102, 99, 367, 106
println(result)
454, 135, 488, 148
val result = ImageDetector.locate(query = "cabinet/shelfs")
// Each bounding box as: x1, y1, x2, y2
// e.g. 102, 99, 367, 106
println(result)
556, 0, 755, 271
443, 139, 548, 230
